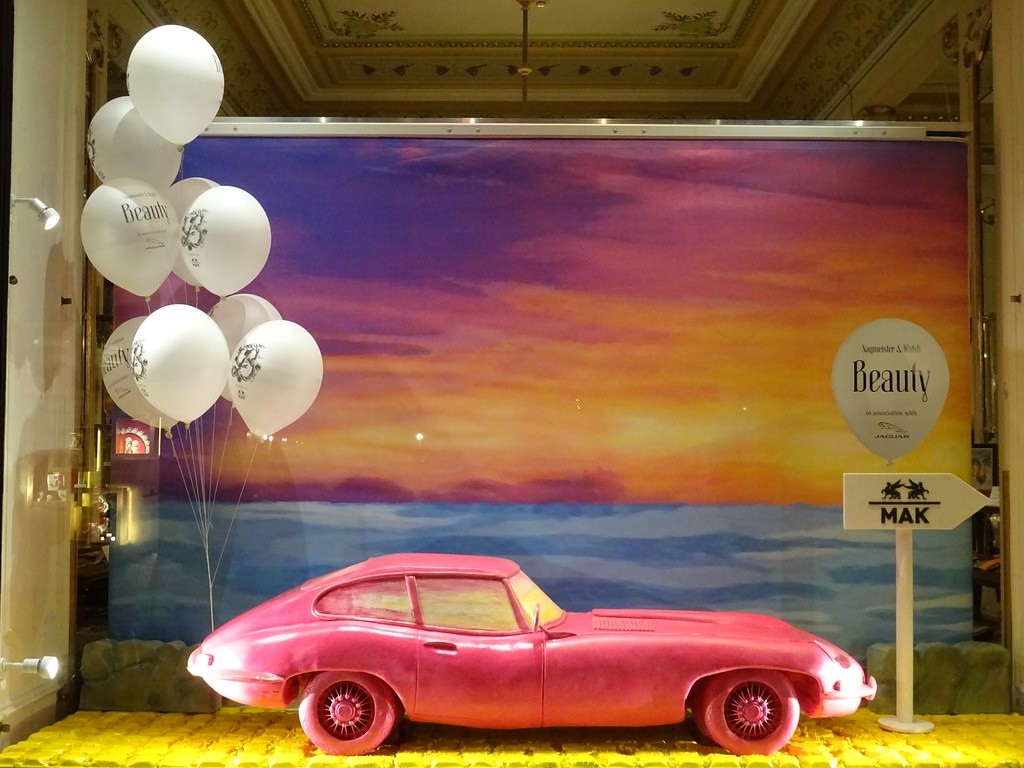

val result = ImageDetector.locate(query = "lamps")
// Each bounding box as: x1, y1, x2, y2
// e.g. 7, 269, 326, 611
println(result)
10, 192, 61, 230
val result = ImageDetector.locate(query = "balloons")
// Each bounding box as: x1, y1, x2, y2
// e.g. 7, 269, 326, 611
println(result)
82, 26, 323, 439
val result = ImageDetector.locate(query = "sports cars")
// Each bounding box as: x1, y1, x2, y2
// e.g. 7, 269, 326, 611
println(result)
184, 554, 879, 757
